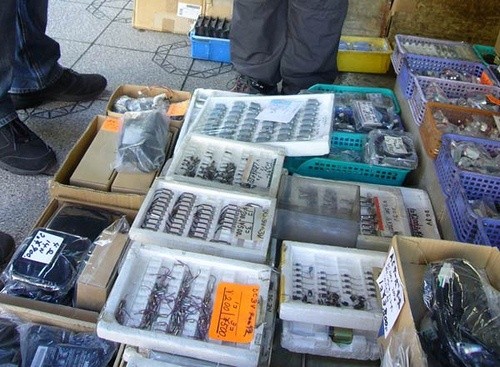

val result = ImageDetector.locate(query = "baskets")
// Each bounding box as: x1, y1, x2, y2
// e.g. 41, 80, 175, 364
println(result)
287, 84, 411, 184
390, 35, 500, 249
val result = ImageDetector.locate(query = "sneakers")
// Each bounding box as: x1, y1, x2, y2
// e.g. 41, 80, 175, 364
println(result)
226, 74, 278, 95
0, 117, 56, 176
11, 66, 107, 108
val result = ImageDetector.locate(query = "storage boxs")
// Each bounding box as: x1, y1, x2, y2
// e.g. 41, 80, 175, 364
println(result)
0, 0, 500, 367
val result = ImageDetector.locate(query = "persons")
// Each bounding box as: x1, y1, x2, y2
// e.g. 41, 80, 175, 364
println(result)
0, 0, 107, 177
230, 0, 348, 94
0, 231, 16, 265
